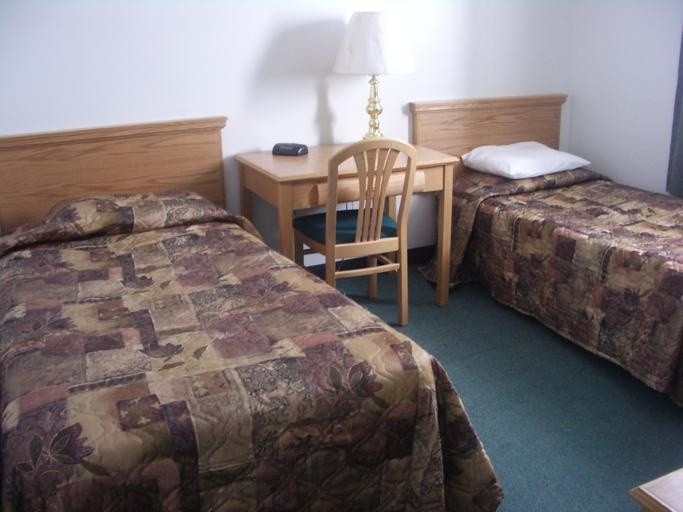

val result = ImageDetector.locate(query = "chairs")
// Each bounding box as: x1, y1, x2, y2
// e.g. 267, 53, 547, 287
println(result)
292, 137, 419, 326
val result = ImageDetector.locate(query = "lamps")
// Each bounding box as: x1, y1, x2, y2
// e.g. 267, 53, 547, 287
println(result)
331, 11, 403, 140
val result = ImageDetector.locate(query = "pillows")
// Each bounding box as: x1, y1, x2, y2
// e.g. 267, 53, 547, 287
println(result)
460, 141, 591, 180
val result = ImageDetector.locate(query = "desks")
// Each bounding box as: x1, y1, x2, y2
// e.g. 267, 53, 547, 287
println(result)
234, 139, 459, 307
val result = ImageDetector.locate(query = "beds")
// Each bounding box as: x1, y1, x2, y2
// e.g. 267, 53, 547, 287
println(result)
408, 93, 683, 408
0, 116, 503, 512
